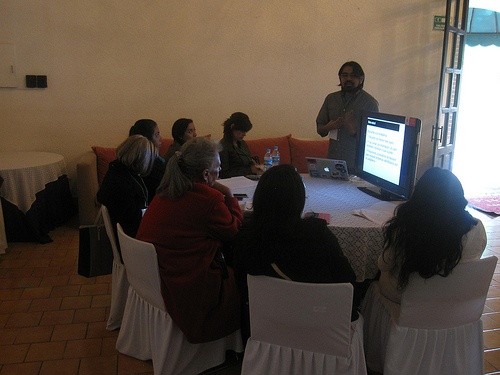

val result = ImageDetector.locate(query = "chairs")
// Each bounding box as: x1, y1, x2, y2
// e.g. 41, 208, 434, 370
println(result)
359, 255, 499, 375
114, 222, 244, 375
240, 274, 369, 375
101, 204, 128, 332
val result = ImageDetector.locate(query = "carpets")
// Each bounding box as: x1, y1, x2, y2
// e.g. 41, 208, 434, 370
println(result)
467, 195, 500, 215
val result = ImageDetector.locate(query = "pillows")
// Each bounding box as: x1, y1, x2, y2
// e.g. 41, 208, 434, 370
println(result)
92, 146, 117, 187
159, 134, 211, 155
289, 136, 329, 173
245, 134, 291, 166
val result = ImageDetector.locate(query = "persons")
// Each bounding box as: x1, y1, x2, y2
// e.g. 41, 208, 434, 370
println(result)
378, 167, 487, 304
316, 61, 379, 175
95, 112, 362, 341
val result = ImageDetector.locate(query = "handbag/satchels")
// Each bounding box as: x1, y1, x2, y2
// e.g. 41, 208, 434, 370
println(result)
78, 207, 112, 278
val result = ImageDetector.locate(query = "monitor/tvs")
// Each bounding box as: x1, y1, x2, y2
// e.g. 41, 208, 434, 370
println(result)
357, 112, 422, 201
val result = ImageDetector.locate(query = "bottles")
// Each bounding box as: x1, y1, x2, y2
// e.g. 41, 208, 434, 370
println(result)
272, 146, 280, 166
263, 149, 272, 172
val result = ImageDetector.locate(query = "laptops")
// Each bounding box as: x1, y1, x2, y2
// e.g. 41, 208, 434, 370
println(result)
305, 157, 357, 181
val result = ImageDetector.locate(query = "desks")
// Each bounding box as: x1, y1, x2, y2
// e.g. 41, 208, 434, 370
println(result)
218, 172, 407, 284
0, 151, 75, 245
